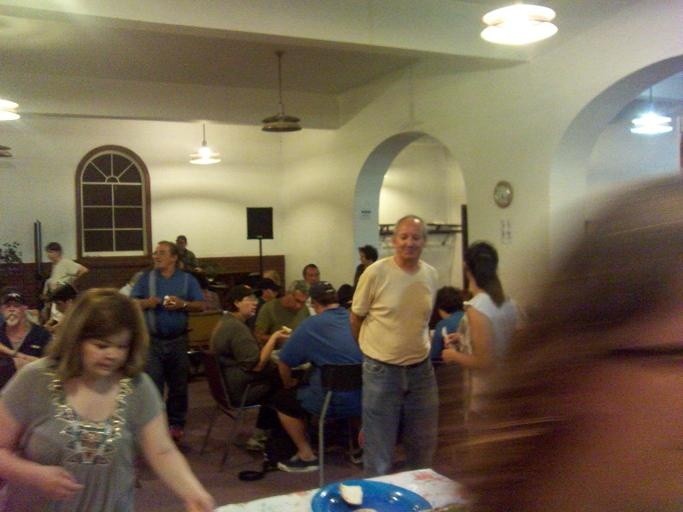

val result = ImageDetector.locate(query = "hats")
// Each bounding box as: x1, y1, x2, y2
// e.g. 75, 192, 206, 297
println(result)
308, 280, 336, 298
45, 284, 77, 303
257, 278, 282, 292
228, 283, 264, 300
0, 287, 26, 306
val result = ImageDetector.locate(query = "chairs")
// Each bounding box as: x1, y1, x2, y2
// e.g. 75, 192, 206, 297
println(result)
279, 363, 362, 488
430, 361, 469, 467
198, 350, 276, 473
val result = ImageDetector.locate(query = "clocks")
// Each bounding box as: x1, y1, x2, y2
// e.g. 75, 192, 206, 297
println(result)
494, 181, 513, 207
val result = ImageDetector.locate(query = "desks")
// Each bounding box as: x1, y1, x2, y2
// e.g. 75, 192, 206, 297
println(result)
186, 309, 227, 378
210, 468, 474, 512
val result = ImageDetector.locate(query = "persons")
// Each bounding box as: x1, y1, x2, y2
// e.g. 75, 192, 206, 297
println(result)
431, 285, 466, 361
0, 242, 91, 392
349, 216, 439, 477
427, 175, 683, 512
440, 242, 525, 420
117, 271, 145, 297
0, 287, 218, 512
130, 240, 214, 441
176, 235, 199, 272
210, 245, 382, 473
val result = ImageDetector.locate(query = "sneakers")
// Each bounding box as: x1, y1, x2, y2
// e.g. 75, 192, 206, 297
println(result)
245, 433, 268, 451
348, 449, 365, 465
277, 453, 320, 474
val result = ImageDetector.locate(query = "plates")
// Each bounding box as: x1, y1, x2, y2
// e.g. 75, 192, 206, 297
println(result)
311, 478, 433, 512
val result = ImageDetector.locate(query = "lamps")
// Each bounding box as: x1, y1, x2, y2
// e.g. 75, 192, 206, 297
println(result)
479, 1, 559, 46
188, 122, 221, 166
630, 84, 672, 135
262, 50, 303, 132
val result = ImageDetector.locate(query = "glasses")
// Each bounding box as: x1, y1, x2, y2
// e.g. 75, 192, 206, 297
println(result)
153, 251, 172, 255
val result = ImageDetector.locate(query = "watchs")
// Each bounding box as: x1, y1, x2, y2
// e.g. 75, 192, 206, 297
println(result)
183, 301, 188, 310
